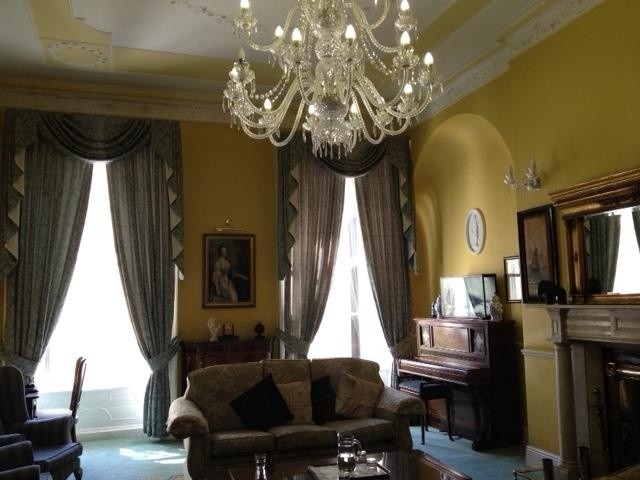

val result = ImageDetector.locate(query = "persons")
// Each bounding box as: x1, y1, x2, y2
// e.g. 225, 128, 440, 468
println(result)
213, 245, 249, 304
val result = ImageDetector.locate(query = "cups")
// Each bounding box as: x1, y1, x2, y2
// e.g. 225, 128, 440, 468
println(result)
358, 450, 378, 475
254, 470, 267, 480
254, 452, 266, 470
336, 431, 362, 475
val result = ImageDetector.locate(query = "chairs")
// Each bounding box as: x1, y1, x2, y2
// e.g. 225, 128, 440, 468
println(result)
0, 364, 30, 435
0, 415, 84, 480
0, 440, 41, 480
36, 355, 88, 444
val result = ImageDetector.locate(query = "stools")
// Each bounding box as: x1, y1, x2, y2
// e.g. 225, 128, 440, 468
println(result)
396, 375, 455, 444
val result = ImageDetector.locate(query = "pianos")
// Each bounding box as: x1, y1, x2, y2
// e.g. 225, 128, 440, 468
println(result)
396, 318, 520, 451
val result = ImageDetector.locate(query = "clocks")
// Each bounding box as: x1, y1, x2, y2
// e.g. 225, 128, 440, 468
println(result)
464, 208, 487, 254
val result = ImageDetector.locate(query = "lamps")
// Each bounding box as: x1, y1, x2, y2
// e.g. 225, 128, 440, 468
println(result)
222, 0, 444, 161
504, 157, 544, 192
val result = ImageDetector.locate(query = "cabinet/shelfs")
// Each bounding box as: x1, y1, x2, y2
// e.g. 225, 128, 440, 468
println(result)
180, 337, 276, 398
24, 394, 38, 419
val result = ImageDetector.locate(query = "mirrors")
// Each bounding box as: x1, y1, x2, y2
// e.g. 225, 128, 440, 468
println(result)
548, 167, 640, 305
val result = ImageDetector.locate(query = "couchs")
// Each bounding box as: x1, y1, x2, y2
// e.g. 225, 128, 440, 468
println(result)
164, 357, 427, 480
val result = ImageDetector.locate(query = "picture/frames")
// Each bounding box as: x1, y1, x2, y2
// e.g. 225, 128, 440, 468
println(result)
503, 256, 523, 305
516, 203, 560, 303
201, 232, 258, 310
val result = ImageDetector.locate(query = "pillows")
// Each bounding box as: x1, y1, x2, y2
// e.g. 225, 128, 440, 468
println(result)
311, 374, 351, 425
275, 380, 314, 425
229, 373, 295, 432
335, 369, 382, 419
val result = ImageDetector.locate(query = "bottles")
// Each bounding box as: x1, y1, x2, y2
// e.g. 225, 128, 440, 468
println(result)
435, 298, 442, 318
255, 321, 265, 336
489, 294, 504, 321
